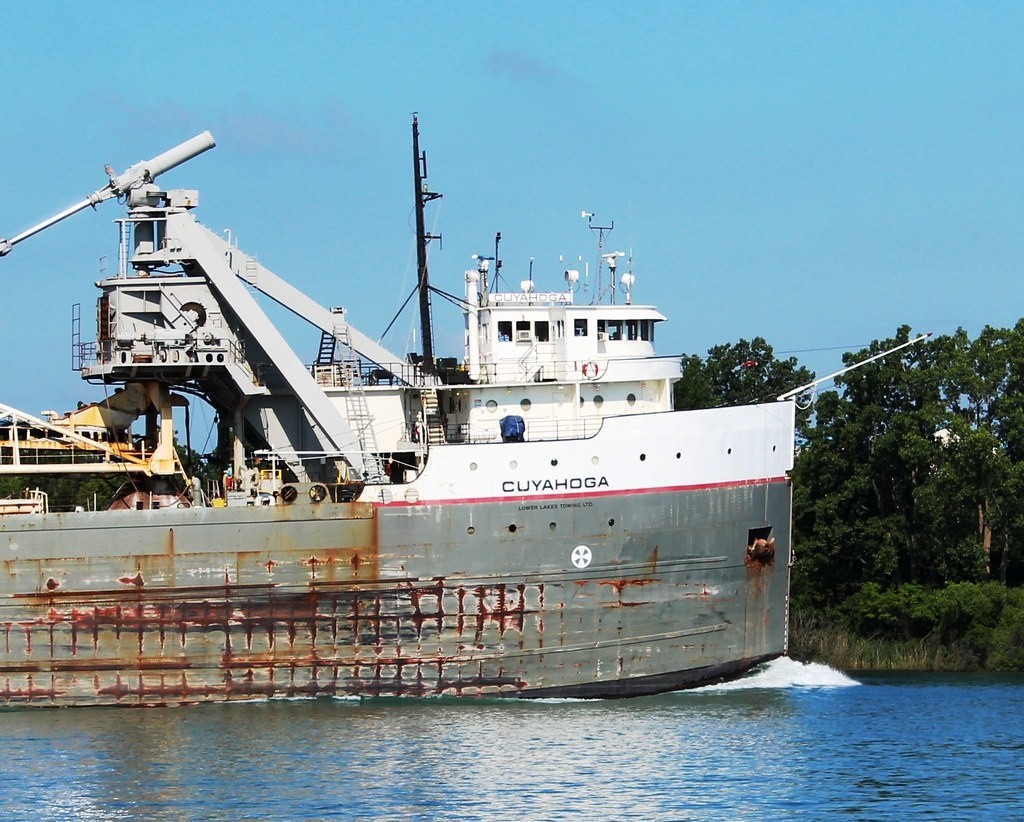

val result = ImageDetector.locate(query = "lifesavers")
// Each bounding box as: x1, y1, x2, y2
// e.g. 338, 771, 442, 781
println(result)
582, 361, 598, 379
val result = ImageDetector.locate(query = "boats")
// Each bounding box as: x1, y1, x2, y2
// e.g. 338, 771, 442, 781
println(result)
0, 102, 800, 709
